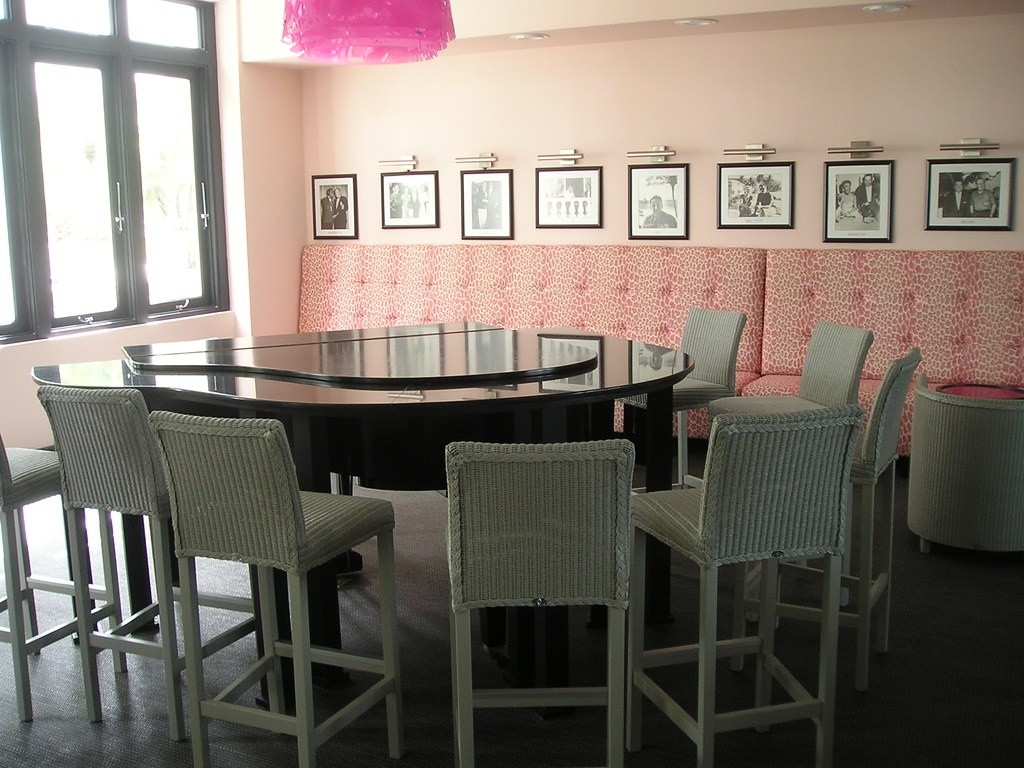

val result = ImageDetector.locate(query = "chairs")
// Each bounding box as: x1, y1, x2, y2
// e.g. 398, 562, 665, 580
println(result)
148, 410, 407, 768
708, 319, 874, 622
41, 386, 259, 741
445, 441, 634, 768
730, 349, 922, 690
613, 307, 747, 496
0, 417, 83, 723
632, 403, 863, 768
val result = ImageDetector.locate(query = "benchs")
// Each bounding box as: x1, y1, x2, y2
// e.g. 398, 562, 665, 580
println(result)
299, 246, 1024, 456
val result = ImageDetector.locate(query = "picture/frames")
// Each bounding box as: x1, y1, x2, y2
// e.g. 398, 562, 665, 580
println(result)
926, 157, 1015, 231
717, 160, 795, 229
311, 174, 358, 240
536, 166, 604, 228
824, 158, 895, 243
460, 168, 514, 240
381, 170, 440, 228
628, 164, 689, 240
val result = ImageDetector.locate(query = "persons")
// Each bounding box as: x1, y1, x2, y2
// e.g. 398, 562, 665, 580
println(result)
836, 174, 879, 224
740, 185, 781, 217
320, 189, 348, 230
475, 181, 499, 229
942, 178, 997, 217
546, 181, 592, 217
645, 343, 673, 371
643, 196, 678, 228
389, 183, 429, 219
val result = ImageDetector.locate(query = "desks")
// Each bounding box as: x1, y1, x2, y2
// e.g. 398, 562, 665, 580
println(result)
32, 321, 695, 712
